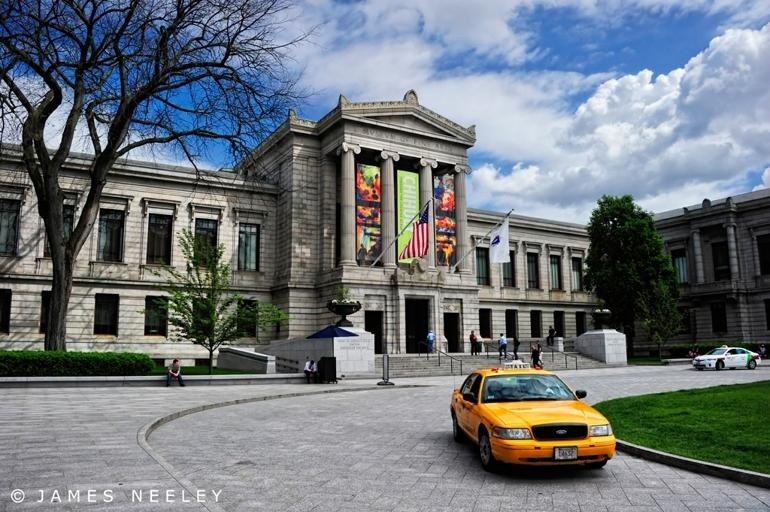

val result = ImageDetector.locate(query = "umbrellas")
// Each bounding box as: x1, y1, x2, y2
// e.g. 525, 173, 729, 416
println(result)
305, 325, 359, 356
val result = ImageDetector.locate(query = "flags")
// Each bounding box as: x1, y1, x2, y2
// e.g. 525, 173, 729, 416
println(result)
489, 216, 511, 264
398, 204, 429, 260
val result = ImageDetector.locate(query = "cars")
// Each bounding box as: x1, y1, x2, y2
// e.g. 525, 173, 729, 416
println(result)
691, 344, 763, 374
449, 357, 619, 472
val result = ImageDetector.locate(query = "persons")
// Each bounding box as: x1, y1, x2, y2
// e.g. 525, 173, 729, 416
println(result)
470, 330, 478, 355
497, 333, 507, 360
538, 347, 544, 366
304, 360, 318, 384
547, 324, 558, 346
426, 330, 436, 352
530, 345, 543, 369
513, 335, 520, 360
537, 340, 542, 351
167, 359, 185, 387
756, 344, 768, 357
